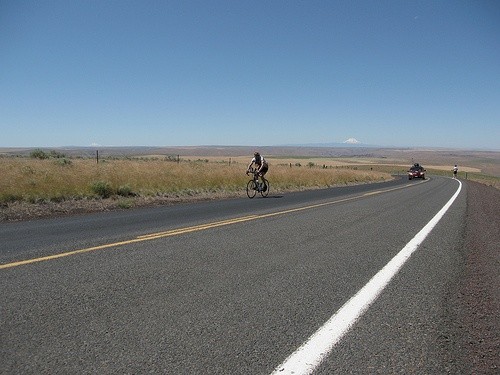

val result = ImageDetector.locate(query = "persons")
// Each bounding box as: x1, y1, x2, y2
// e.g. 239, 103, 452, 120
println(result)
451, 165, 459, 179
247, 151, 268, 192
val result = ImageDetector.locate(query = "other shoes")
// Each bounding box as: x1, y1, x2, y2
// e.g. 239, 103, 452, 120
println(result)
263, 186, 267, 191
253, 187, 258, 190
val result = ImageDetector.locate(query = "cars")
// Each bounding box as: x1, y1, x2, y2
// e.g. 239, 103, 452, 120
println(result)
407, 163, 426, 180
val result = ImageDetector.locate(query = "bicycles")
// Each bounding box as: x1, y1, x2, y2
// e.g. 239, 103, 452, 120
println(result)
246, 170, 269, 198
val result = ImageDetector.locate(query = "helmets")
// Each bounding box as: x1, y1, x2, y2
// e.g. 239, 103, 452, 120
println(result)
254, 151, 260, 157
